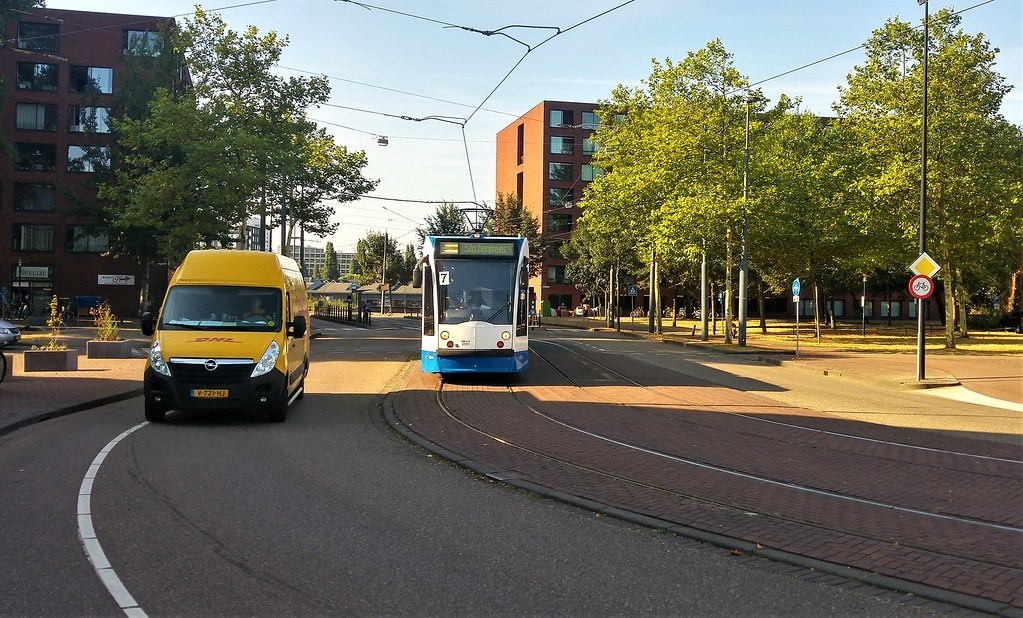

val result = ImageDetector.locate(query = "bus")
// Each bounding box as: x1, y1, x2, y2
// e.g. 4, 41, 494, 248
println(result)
412, 208, 529, 374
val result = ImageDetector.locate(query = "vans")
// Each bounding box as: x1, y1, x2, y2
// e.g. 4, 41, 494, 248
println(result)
143, 249, 311, 423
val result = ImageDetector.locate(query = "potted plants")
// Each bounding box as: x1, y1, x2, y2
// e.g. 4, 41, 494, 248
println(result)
23, 295, 78, 372
86, 298, 132, 359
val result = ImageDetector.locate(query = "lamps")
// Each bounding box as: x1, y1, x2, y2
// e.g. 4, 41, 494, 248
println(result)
377, 135, 388, 146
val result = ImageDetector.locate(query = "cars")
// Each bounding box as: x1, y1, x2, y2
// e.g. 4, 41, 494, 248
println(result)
0, 319, 22, 348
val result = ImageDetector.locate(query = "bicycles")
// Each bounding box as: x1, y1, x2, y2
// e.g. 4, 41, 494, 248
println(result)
63, 305, 75, 322
662, 306, 720, 320
0, 349, 6, 383
630, 306, 645, 318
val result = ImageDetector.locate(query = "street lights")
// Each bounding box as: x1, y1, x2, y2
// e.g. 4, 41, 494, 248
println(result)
916, 0, 929, 382
738, 96, 761, 348
